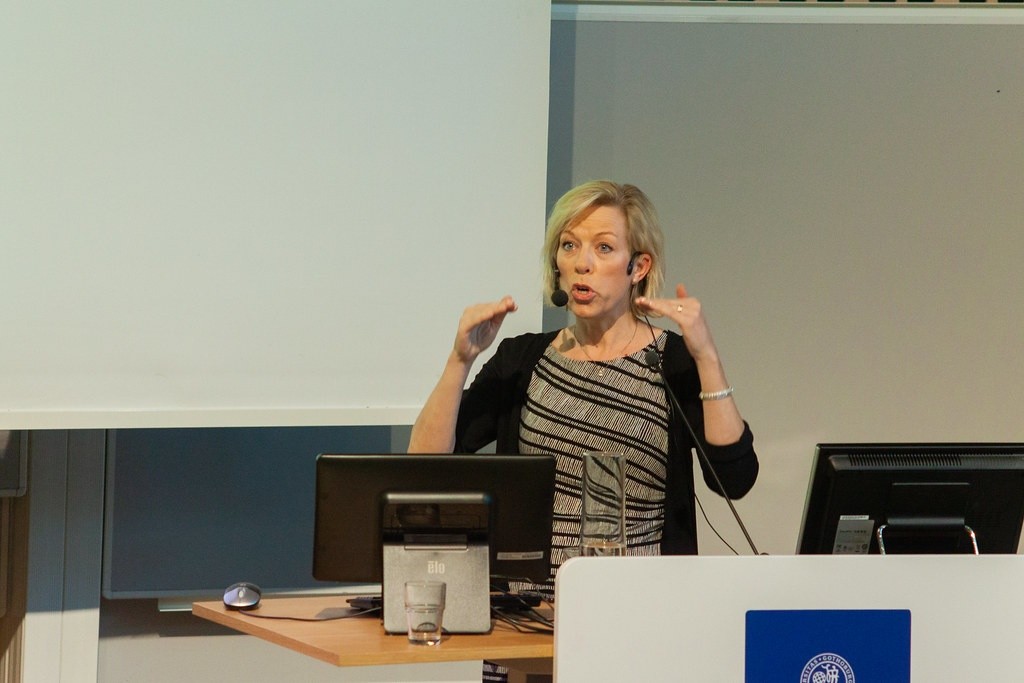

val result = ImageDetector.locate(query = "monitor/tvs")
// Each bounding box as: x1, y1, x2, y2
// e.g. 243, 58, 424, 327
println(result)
795, 442, 1024, 554
312, 454, 555, 584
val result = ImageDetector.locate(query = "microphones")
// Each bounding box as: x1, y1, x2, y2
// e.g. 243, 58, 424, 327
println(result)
551, 266, 568, 307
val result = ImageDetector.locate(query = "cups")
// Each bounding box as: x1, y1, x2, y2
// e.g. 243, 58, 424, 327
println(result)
405, 581, 446, 645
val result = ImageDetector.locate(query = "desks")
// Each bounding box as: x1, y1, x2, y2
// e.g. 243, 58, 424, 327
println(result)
192, 592, 555, 683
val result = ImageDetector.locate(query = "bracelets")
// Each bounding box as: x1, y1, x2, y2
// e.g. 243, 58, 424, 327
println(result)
698, 384, 734, 401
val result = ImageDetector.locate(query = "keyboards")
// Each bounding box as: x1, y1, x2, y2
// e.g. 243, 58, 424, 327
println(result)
346, 593, 541, 612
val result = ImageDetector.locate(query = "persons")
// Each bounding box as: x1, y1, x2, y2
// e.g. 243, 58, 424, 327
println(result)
408, 178, 760, 683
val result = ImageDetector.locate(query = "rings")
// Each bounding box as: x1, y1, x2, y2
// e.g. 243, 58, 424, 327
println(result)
676, 302, 683, 313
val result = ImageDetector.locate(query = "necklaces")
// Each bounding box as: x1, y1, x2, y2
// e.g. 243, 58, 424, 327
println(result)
573, 316, 638, 376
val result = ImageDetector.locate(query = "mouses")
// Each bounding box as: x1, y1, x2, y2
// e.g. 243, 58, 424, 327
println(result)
224, 581, 262, 610
